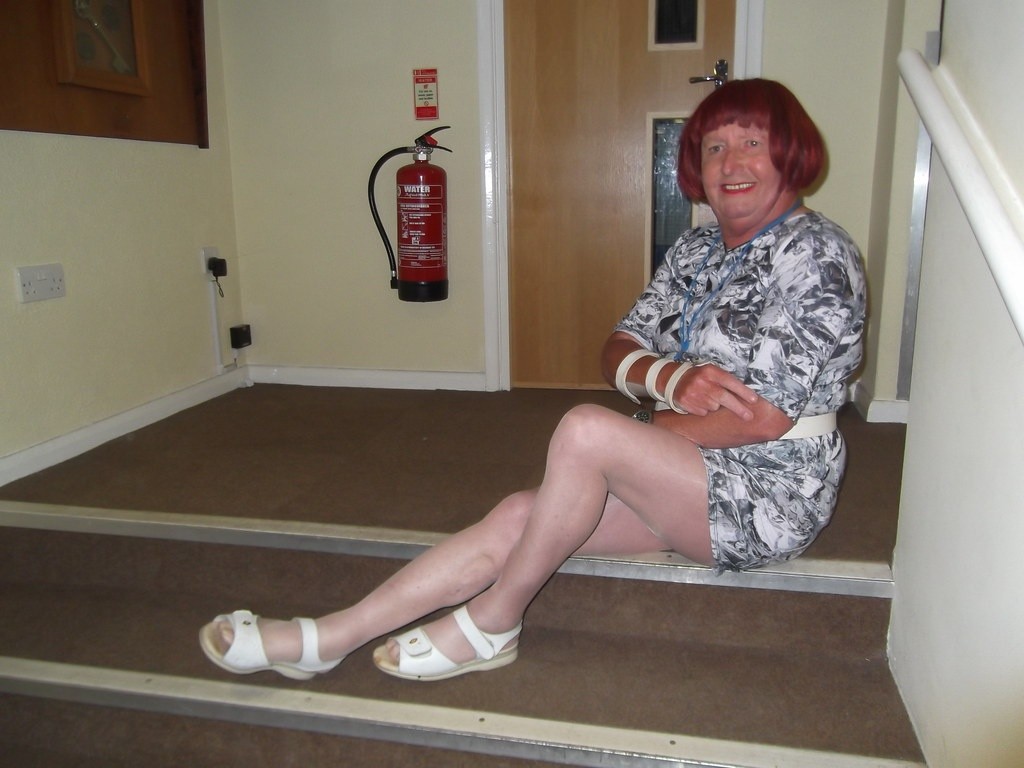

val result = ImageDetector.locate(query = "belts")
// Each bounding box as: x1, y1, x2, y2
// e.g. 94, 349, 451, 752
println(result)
778, 411, 838, 439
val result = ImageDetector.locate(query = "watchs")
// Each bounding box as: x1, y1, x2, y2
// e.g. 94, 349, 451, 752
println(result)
632, 408, 655, 423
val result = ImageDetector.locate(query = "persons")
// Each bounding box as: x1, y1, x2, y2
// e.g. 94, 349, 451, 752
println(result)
199, 78, 866, 681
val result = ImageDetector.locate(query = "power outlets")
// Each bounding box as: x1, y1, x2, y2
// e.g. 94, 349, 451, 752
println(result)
202, 246, 220, 272
15, 262, 68, 302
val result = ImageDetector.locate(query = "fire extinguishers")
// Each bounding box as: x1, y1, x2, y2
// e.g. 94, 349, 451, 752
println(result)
367, 126, 453, 303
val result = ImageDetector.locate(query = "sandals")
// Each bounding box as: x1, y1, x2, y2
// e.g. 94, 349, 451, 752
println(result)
372, 604, 523, 681
198, 610, 347, 681
